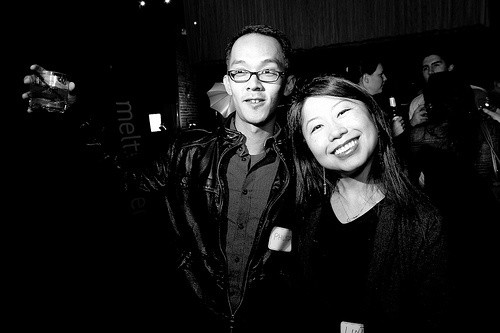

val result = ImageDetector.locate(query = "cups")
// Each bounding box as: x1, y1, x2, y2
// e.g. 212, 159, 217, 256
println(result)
30, 71, 69, 112
476, 91, 496, 119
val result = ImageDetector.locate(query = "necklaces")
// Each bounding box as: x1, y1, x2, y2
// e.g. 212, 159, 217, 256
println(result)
337, 183, 381, 222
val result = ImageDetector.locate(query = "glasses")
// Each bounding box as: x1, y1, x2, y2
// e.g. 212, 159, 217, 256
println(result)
227, 69, 286, 83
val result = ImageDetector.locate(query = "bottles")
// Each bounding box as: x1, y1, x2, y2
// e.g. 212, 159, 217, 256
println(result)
389, 97, 399, 116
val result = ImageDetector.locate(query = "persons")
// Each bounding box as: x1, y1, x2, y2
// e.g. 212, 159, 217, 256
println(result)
347, 59, 406, 136
22, 24, 296, 333
287, 75, 444, 333
409, 50, 500, 186
